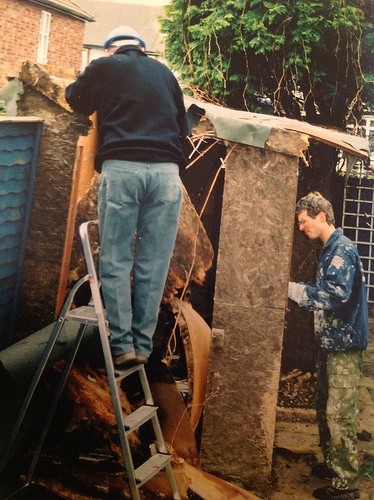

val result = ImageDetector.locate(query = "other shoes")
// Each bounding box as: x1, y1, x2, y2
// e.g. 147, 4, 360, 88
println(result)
133, 355, 148, 364
311, 461, 339, 481
313, 485, 361, 500
114, 352, 135, 366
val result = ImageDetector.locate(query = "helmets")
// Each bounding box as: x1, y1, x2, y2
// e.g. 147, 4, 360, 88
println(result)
103, 26, 145, 50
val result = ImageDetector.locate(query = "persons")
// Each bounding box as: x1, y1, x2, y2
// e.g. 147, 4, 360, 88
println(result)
65, 25, 190, 367
288, 190, 370, 500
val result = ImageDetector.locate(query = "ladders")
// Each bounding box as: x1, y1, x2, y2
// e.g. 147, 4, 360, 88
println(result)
0, 220, 180, 500
